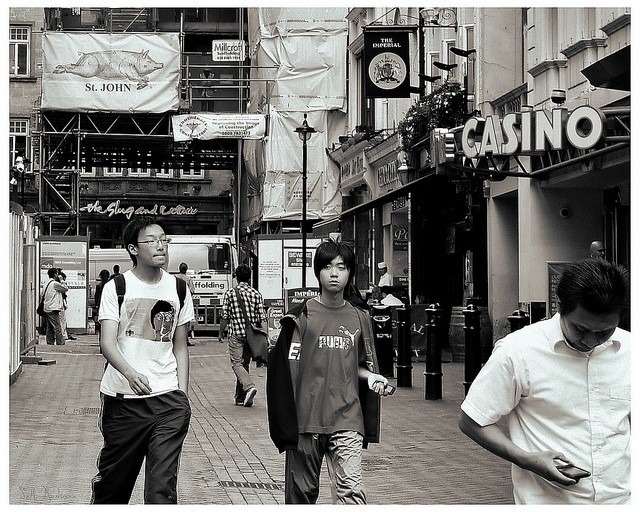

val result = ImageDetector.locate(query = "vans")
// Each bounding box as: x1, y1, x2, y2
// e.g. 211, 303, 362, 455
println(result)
88, 248, 134, 309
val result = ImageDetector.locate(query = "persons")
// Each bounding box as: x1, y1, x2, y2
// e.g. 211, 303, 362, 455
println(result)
458, 257, 631, 504
172, 262, 196, 346
56, 268, 77, 340
378, 262, 392, 287
86, 216, 196, 504
58, 274, 69, 340
109, 263, 122, 280
218, 264, 269, 407
590, 241, 605, 258
93, 268, 110, 353
267, 236, 388, 503
42, 267, 69, 345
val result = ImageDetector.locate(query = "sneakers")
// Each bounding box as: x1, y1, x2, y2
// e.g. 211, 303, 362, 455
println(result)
244, 387, 258, 407
187, 341, 195, 346
236, 398, 244, 405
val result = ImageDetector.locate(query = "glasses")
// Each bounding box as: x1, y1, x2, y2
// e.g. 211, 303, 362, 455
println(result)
137, 237, 173, 246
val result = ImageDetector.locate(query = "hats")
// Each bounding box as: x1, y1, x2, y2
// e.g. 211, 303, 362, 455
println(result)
378, 262, 387, 269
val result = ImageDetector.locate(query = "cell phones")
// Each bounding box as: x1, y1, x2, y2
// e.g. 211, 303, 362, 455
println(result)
372, 380, 396, 396
553, 458, 591, 479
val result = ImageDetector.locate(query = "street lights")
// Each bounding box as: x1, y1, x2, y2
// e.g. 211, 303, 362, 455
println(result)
294, 113, 317, 288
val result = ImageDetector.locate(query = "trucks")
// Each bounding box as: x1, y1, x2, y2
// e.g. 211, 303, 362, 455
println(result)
165, 235, 254, 331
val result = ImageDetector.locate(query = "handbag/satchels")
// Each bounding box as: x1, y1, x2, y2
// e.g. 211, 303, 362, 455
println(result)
245, 323, 270, 365
37, 302, 46, 318
